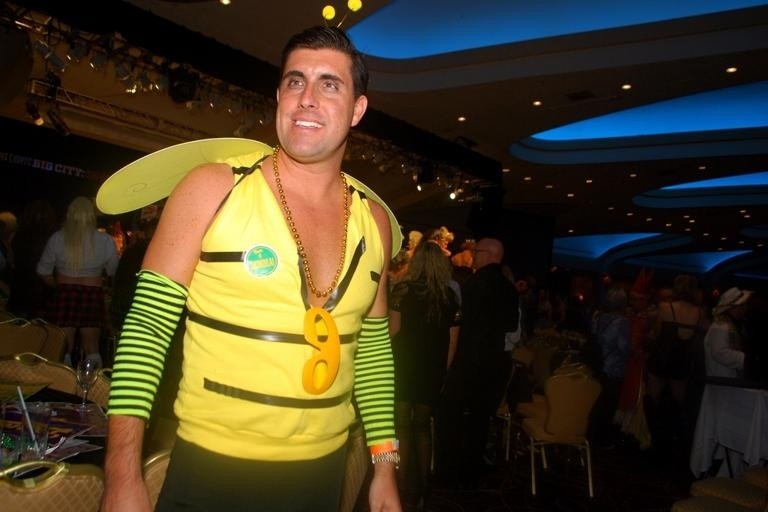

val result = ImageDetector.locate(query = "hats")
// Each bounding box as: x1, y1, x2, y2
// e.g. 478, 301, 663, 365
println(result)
712, 286, 754, 314
432, 226, 454, 241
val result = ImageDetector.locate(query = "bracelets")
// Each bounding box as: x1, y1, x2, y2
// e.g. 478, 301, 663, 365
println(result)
368, 450, 400, 464
364, 441, 399, 454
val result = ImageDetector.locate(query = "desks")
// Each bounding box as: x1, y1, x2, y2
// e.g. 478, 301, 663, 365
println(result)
703, 383, 767, 479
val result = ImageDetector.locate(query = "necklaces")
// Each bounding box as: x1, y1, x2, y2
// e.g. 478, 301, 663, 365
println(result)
271, 140, 352, 299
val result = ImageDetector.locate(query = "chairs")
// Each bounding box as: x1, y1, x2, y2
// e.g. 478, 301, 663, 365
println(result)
515, 361, 603, 496
0, 310, 173, 511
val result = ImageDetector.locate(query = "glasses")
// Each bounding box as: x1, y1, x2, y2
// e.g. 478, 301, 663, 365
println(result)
471, 247, 489, 255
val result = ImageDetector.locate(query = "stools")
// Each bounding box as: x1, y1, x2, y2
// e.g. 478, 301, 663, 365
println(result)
667, 464, 768, 510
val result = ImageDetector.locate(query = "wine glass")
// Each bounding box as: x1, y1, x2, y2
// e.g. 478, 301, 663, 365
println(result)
76, 360, 97, 413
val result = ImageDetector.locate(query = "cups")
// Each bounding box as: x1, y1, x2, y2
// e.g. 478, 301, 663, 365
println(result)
20, 407, 51, 461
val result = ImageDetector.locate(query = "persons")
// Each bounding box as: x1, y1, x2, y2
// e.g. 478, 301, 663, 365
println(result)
386, 216, 768, 511
0, 191, 161, 364
89, 22, 406, 512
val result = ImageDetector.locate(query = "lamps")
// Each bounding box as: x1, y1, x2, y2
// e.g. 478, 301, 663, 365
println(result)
23, 96, 73, 137
